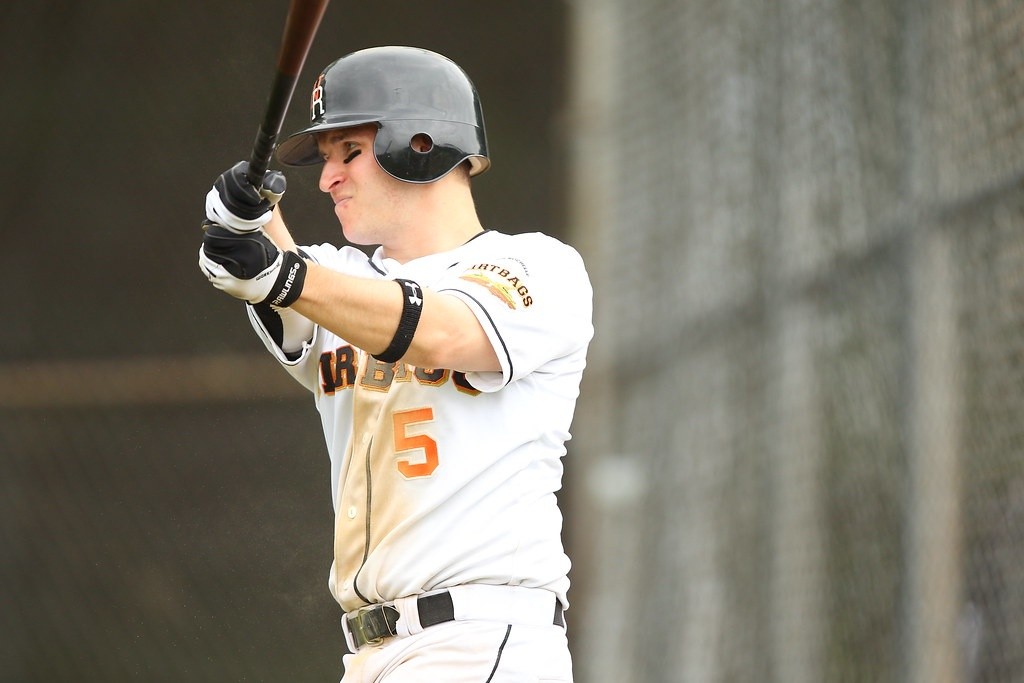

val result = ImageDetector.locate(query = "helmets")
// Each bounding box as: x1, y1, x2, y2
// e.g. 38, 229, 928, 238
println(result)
274, 46, 492, 184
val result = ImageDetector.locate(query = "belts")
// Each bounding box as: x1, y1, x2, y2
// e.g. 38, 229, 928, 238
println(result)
347, 591, 565, 648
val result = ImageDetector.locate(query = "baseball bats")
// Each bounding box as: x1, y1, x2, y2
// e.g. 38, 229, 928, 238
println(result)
248, 0, 329, 191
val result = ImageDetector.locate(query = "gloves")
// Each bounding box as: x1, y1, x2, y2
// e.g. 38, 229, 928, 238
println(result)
206, 160, 288, 234
198, 219, 307, 307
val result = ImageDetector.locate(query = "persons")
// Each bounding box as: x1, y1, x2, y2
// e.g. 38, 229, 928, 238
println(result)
198, 46, 594, 683
